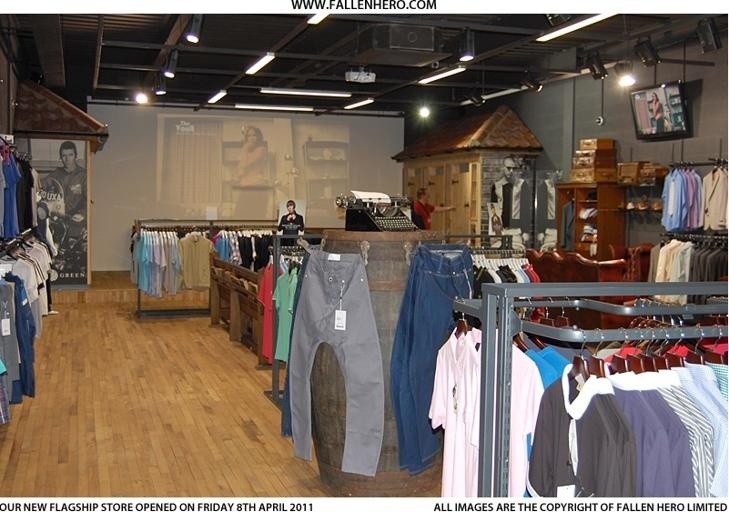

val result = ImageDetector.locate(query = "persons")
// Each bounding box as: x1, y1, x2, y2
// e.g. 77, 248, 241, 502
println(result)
490, 156, 531, 249
233, 126, 269, 219
413, 188, 457, 231
277, 201, 305, 245
537, 179, 557, 245
491, 203, 501, 236
47, 141, 87, 272
652, 93, 664, 133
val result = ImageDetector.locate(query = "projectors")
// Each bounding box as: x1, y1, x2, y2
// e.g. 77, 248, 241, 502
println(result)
345, 71, 376, 83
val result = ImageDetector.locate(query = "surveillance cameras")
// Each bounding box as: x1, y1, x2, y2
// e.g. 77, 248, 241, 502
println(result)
432, 61, 439, 68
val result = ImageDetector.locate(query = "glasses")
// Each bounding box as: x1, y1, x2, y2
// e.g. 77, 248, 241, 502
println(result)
506, 167, 516, 170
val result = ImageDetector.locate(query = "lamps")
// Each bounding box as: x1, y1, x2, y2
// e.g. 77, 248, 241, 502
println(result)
186, 14, 203, 44
153, 69, 168, 95
163, 47, 179, 79
135, 91, 148, 105
416, 14, 722, 116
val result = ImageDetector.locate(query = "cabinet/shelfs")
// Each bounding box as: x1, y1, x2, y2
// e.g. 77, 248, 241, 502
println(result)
555, 181, 625, 259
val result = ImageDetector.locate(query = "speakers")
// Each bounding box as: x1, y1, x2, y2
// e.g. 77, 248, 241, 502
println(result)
378, 23, 435, 52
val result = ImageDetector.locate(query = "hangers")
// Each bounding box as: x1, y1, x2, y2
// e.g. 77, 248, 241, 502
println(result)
469, 246, 530, 269
0, 228, 36, 266
134, 219, 277, 236
656, 229, 728, 251
268, 243, 308, 271
447, 295, 569, 349
667, 159, 728, 171
569, 303, 728, 381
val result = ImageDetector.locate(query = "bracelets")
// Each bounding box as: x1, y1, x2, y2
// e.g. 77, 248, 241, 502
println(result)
448, 207, 449, 210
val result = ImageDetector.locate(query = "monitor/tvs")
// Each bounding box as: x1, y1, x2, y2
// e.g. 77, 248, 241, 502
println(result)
627, 79, 692, 141
